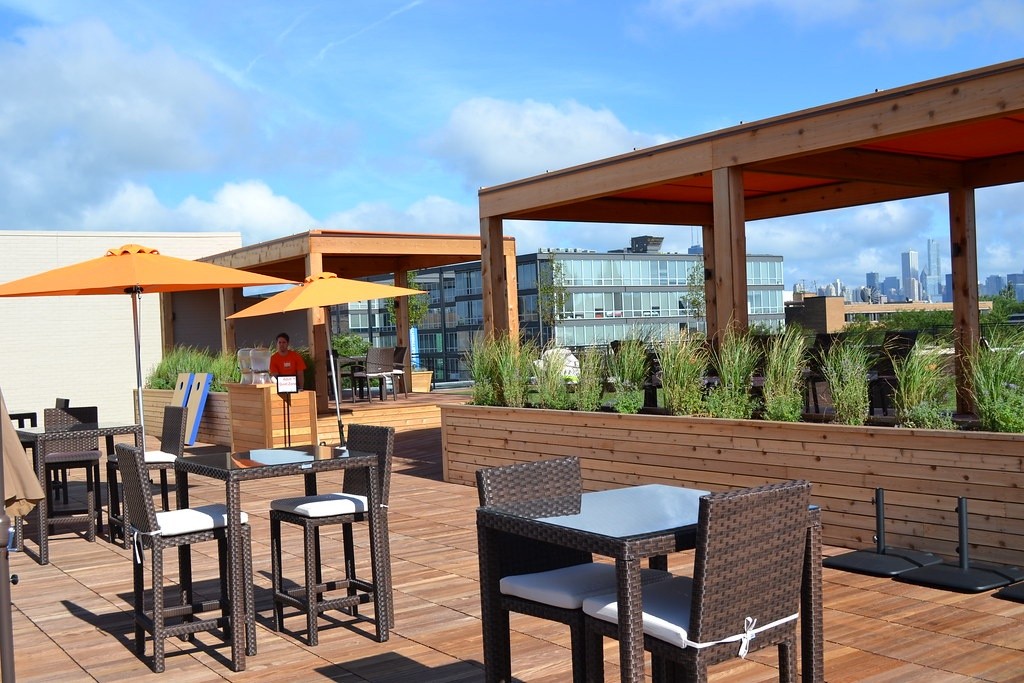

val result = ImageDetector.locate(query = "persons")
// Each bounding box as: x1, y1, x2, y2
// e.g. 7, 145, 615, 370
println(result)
269, 333, 307, 389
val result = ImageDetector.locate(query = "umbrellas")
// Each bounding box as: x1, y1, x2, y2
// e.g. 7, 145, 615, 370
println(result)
226, 273, 429, 458
1, 245, 303, 485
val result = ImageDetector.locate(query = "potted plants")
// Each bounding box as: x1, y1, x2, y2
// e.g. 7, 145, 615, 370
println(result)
412, 367, 435, 392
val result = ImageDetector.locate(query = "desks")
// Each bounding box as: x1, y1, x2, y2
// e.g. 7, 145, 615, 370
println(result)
329, 356, 387, 402
173, 444, 391, 673
476, 483, 827, 683
16, 422, 145, 565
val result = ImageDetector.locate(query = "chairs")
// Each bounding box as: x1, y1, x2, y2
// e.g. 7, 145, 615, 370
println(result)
352, 347, 397, 405
584, 478, 813, 683
42, 406, 104, 544
862, 329, 918, 412
269, 424, 397, 644
739, 334, 789, 414
326, 350, 357, 398
115, 441, 258, 677
474, 455, 674, 683
363, 346, 408, 399
611, 338, 647, 359
9, 412, 36, 475
105, 404, 188, 548
22, 396, 70, 509
793, 332, 848, 413
